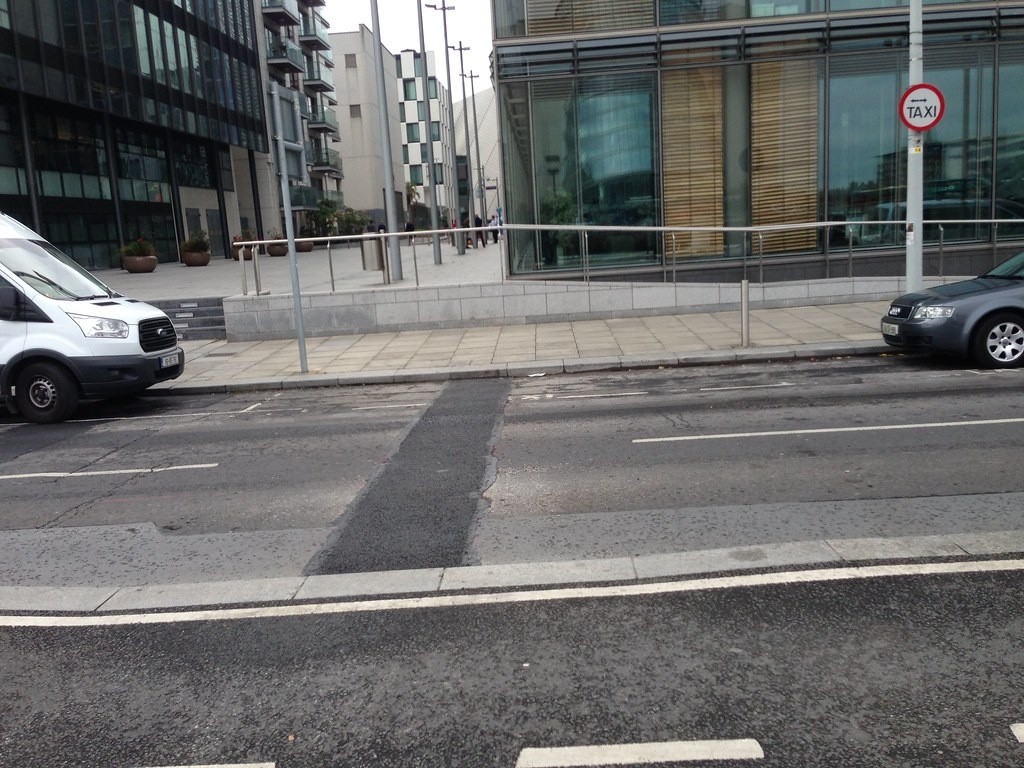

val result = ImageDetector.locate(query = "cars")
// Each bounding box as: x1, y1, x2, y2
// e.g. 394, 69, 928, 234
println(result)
862, 199, 1023, 244
879, 249, 1024, 368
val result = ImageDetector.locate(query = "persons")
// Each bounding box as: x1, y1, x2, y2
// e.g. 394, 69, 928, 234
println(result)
325, 211, 504, 252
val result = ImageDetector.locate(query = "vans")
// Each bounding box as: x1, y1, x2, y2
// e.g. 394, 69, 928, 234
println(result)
1, 209, 185, 423
